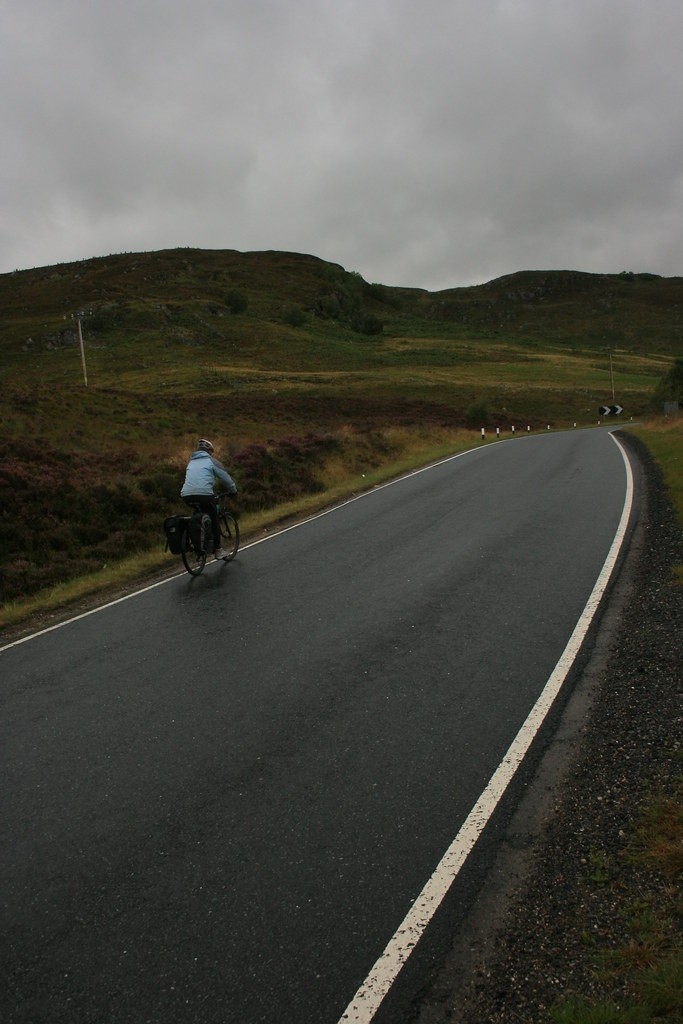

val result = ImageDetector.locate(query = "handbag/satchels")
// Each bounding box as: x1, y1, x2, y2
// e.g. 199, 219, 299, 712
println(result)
163, 514, 214, 555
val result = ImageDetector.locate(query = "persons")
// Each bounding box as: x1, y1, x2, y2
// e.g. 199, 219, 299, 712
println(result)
181, 439, 237, 560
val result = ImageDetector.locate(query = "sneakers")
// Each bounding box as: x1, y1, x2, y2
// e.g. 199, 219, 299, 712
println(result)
215, 548, 228, 559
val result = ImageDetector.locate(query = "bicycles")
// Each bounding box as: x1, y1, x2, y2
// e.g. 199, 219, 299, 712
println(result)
180, 493, 241, 577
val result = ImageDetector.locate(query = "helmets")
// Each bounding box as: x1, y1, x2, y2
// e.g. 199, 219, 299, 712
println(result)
197, 439, 214, 453
